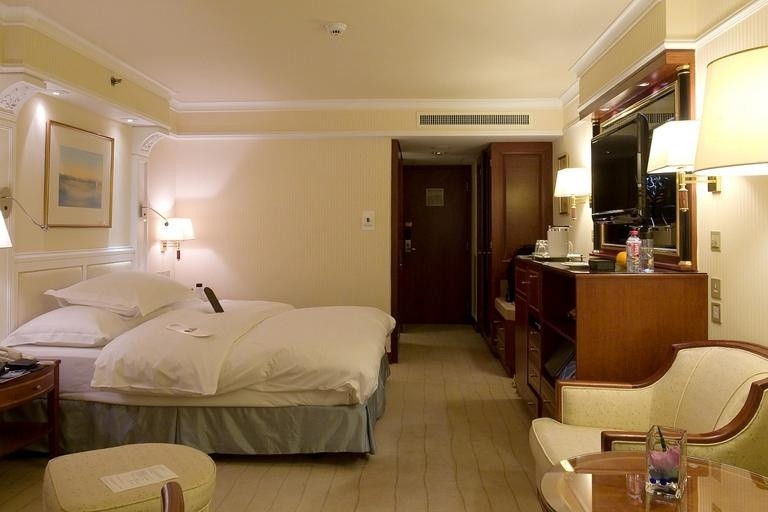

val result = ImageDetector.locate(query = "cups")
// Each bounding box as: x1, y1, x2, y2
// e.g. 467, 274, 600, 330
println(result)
535, 240, 573, 255
642, 239, 654, 273
644, 423, 688, 502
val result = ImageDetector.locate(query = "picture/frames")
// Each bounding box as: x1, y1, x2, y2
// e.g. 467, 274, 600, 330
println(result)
44, 119, 115, 229
557, 153, 570, 215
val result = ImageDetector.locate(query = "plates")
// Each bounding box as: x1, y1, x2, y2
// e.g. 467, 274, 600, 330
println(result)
561, 261, 589, 269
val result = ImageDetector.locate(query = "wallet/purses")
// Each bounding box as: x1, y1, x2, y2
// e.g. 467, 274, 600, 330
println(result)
7, 359, 37, 370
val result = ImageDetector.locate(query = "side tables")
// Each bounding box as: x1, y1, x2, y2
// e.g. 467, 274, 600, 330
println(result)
536, 451, 767, 511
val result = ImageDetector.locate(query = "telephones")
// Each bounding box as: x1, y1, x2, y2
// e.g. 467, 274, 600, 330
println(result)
0, 346, 37, 366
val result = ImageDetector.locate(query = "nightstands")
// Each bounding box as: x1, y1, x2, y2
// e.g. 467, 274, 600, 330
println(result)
0, 359, 61, 458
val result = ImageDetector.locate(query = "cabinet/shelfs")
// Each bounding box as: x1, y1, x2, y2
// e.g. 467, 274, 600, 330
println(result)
513, 254, 708, 421
495, 298, 516, 377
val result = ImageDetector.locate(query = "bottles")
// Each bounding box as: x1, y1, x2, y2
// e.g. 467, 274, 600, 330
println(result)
626, 231, 641, 271
626, 471, 645, 505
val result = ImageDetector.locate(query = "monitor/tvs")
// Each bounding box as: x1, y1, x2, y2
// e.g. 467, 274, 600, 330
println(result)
591, 113, 649, 223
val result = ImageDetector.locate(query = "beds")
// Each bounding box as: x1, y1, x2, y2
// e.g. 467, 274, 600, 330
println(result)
8, 248, 397, 461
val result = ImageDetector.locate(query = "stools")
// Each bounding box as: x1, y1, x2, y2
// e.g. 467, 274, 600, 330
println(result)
42, 443, 217, 512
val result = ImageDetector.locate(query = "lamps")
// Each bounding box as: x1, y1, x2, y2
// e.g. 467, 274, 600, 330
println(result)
693, 46, 768, 172
0, 211, 12, 249
554, 169, 592, 218
646, 119, 720, 211
140, 207, 196, 241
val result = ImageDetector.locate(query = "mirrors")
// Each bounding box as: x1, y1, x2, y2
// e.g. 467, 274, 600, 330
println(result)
597, 79, 685, 258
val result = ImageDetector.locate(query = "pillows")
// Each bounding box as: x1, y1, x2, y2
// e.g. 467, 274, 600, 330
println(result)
45, 271, 193, 318
1, 304, 174, 348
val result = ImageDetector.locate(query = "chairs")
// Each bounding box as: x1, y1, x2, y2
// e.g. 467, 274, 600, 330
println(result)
528, 340, 768, 491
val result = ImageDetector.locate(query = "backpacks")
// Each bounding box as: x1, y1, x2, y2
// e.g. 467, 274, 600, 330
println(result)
505, 245, 535, 302
